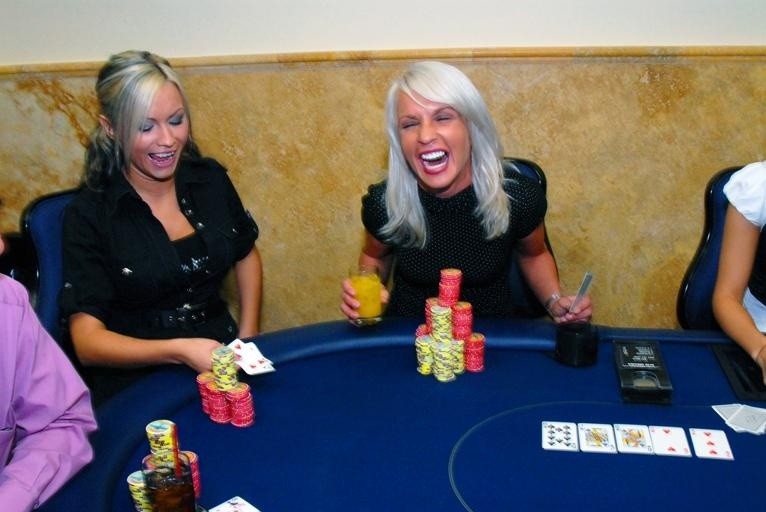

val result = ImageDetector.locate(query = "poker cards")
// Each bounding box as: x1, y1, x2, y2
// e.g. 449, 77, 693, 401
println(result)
541, 421, 735, 461
710, 403, 766, 435
569, 272, 592, 314
226, 338, 276, 375
207, 496, 261, 512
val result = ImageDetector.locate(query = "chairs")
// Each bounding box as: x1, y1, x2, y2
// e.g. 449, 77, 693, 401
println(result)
675, 166, 742, 331
19, 185, 85, 349
499, 157, 559, 321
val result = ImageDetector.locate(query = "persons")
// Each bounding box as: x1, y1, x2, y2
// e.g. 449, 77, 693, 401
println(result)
0, 199, 99, 511
53, 50, 264, 412
710, 157, 766, 387
337, 60, 594, 327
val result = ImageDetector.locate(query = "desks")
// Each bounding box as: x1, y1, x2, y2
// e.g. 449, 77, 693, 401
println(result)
30, 317, 766, 512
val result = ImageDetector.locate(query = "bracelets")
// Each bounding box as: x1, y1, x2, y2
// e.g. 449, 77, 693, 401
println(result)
755, 343, 766, 363
546, 292, 563, 313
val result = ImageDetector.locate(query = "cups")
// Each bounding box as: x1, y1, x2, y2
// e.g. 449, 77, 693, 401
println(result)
142, 451, 194, 509
349, 265, 384, 327
